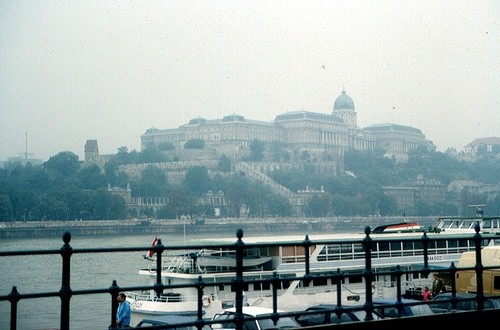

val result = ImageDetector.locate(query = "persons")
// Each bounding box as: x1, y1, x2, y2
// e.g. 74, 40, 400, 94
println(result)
144, 238, 158, 258
115, 293, 130, 327
432, 273, 446, 300
422, 286, 431, 300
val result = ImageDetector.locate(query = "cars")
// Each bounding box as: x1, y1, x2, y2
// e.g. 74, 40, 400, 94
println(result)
212, 306, 303, 329
297, 304, 384, 326
137, 316, 214, 329
430, 292, 499, 314
366, 297, 436, 317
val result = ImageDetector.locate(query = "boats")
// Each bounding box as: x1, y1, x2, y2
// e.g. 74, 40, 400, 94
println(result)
123, 204, 500, 327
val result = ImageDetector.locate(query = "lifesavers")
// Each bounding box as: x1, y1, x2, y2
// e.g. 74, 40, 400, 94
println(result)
372, 284, 375, 293
202, 296, 211, 307
